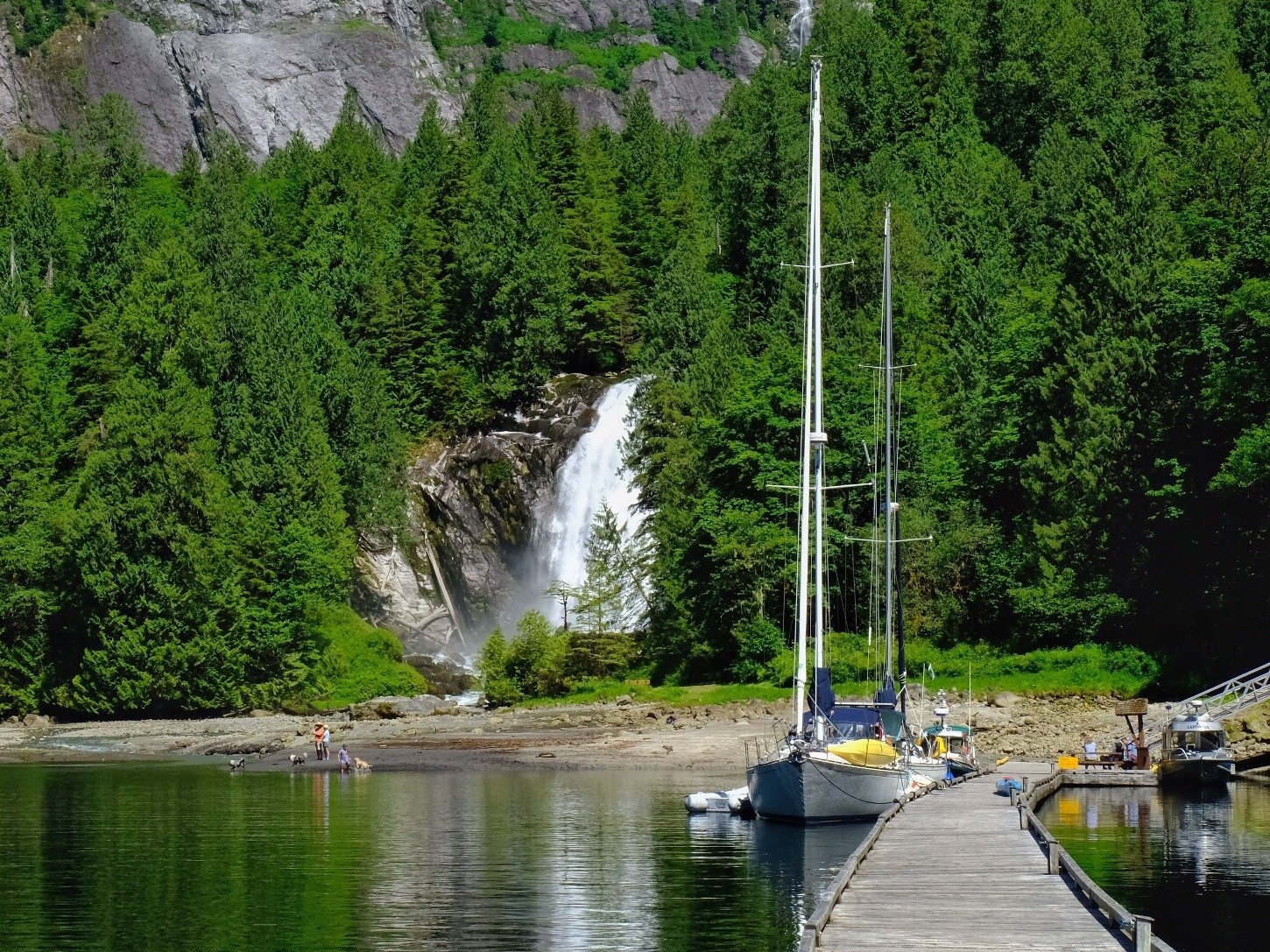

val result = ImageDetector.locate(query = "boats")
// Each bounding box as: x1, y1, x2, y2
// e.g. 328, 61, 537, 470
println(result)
1157, 700, 1235, 786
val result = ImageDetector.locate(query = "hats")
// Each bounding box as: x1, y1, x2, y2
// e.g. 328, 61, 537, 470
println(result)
315, 722, 323, 726
324, 725, 328, 729
1115, 742, 1122, 747
343, 743, 348, 747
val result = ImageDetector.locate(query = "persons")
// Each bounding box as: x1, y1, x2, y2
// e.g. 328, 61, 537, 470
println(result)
1121, 737, 1137, 769
1084, 737, 1099, 774
1102, 741, 1124, 770
320, 725, 330, 760
313, 721, 324, 760
338, 743, 352, 772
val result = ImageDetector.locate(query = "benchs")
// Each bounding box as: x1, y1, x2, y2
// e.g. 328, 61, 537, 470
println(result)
1067, 752, 1124, 775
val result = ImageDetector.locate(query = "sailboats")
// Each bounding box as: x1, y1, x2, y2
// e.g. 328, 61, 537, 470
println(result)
746, 53, 979, 820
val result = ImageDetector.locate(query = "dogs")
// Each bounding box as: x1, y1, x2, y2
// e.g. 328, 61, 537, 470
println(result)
288, 752, 308, 767
353, 757, 373, 769
229, 758, 246, 770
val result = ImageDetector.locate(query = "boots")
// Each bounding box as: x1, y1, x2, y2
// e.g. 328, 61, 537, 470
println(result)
316, 750, 319, 760
319, 750, 323, 760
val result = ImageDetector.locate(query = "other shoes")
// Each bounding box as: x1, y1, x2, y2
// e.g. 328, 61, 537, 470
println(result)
1103, 767, 1107, 770
1108, 767, 1111, 770
